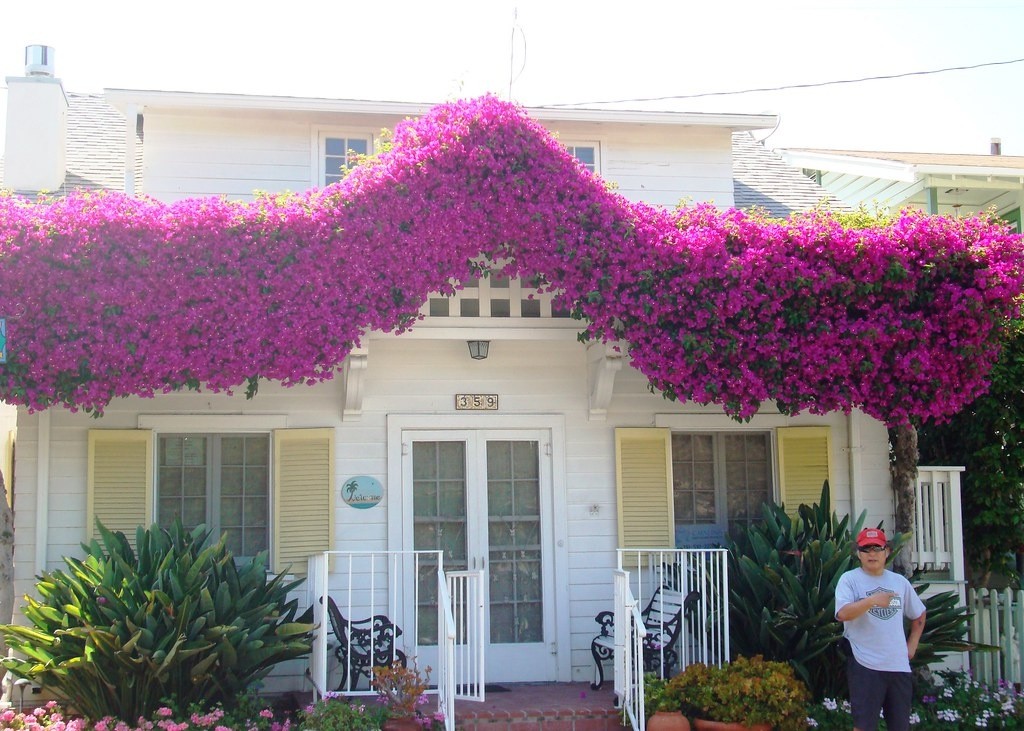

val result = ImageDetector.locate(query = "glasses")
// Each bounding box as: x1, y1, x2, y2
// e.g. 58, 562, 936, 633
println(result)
858, 544, 886, 553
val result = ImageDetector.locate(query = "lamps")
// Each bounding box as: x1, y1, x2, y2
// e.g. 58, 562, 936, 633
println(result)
466, 340, 491, 360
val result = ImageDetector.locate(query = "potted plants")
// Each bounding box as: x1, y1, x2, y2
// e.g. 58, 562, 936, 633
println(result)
370, 656, 433, 731
643, 671, 690, 731
673, 654, 811, 731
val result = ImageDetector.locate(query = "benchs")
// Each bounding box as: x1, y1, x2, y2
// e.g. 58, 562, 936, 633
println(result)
319, 595, 406, 704
590, 585, 701, 706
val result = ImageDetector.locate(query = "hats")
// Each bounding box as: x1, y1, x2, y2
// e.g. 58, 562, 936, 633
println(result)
855, 528, 887, 547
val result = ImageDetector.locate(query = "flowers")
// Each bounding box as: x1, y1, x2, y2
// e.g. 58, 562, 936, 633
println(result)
0, 92, 1024, 430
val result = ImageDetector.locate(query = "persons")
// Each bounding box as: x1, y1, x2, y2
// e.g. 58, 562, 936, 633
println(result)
835, 527, 926, 731
